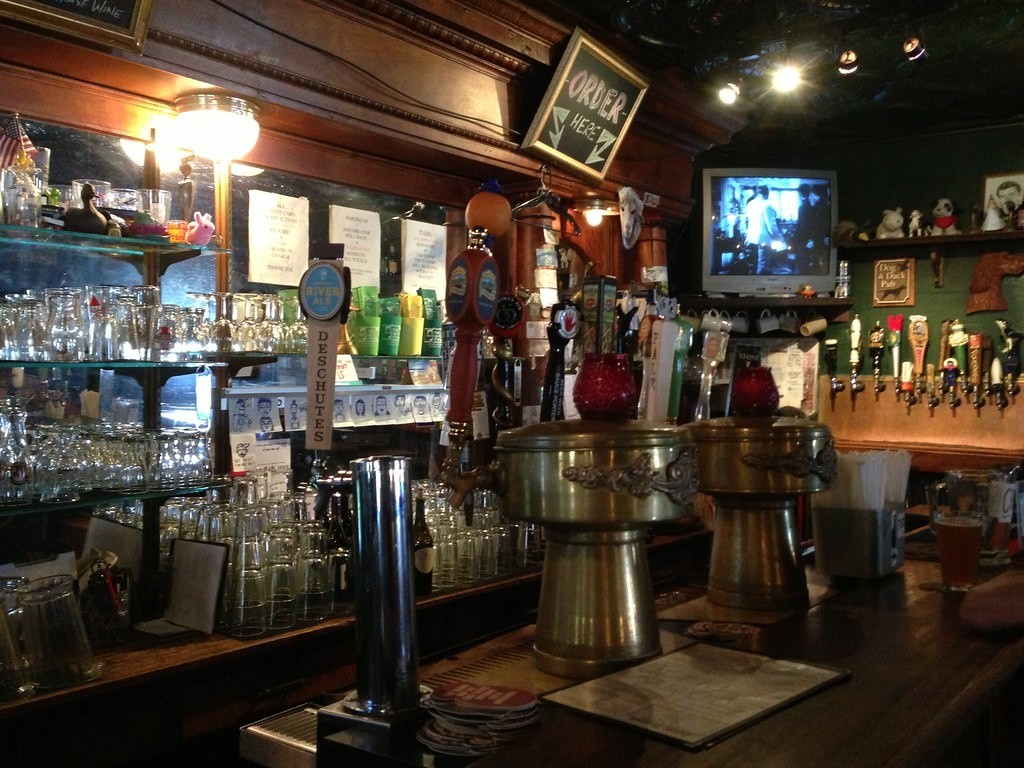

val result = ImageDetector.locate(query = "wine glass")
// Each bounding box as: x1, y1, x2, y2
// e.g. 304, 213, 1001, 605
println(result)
184, 289, 309, 355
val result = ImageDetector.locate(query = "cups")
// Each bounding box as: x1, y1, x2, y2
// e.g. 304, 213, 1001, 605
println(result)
20, 422, 214, 504
355, 315, 444, 357
0, 281, 206, 364
925, 468, 1015, 567
932, 510, 985, 592
409, 479, 545, 593
46, 179, 173, 223
756, 309, 802, 335
800, 309, 828, 337
0, 573, 103, 706
678, 307, 751, 334
92, 479, 332, 640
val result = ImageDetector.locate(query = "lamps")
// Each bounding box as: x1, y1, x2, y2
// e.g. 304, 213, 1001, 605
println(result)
903, 38, 929, 64
572, 195, 620, 227
172, 95, 260, 161
837, 39, 861, 69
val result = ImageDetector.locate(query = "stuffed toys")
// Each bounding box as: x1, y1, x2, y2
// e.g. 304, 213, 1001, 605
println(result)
184, 211, 216, 245
908, 207, 933, 237
928, 199, 961, 236
875, 204, 904, 238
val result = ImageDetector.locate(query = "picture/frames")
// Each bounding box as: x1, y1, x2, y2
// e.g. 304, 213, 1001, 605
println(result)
981, 171, 1024, 220
519, 26, 651, 188
0, 0, 155, 57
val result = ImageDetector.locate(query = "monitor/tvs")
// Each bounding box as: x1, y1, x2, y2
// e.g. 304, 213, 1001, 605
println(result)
702, 168, 838, 297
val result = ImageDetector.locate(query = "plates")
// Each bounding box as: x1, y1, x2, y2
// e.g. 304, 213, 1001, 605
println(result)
342, 684, 435, 717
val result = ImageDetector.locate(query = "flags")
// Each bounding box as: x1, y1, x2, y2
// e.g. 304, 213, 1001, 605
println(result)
1, 112, 40, 172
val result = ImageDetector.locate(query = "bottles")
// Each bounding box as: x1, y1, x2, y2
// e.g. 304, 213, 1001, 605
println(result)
316, 481, 355, 612
408, 497, 434, 595
0, 396, 34, 508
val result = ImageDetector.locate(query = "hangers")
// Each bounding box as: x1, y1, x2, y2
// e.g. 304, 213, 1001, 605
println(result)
511, 164, 582, 236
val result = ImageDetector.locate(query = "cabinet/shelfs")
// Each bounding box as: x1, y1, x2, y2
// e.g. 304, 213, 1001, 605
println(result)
0, 223, 231, 519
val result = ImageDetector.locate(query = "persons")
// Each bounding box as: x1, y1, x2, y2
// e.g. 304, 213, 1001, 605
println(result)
715, 182, 832, 275
